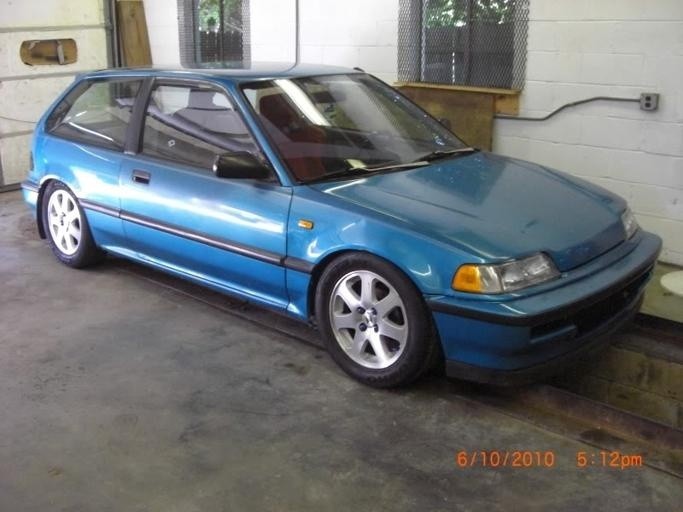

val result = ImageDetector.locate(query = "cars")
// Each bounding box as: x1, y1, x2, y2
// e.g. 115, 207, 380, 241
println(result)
23, 66, 662, 391
